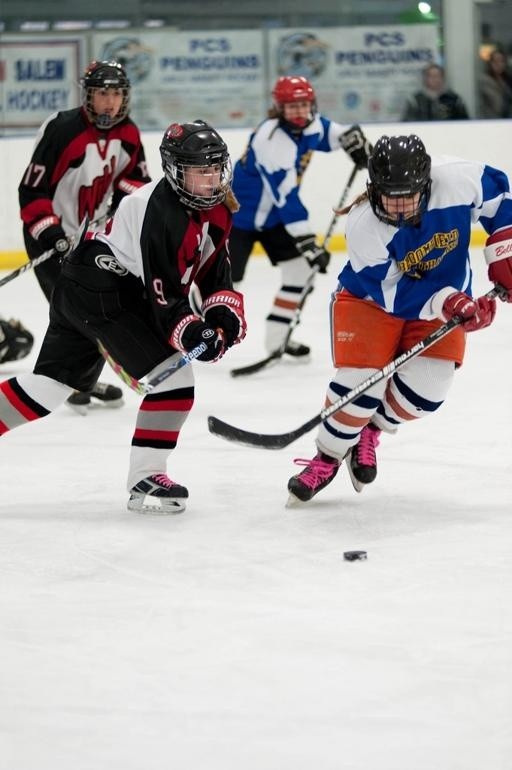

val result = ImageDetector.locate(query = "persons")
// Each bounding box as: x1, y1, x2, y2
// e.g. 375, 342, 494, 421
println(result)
18, 60, 153, 415
285, 134, 512, 511
0, 119, 247, 515
227, 76, 375, 365
480, 49, 512, 119
401, 64, 470, 120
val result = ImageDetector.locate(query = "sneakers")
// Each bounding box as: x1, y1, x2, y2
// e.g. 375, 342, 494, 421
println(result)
130, 472, 188, 497
288, 450, 340, 500
91, 382, 123, 401
284, 341, 309, 356
68, 392, 91, 406
351, 422, 381, 483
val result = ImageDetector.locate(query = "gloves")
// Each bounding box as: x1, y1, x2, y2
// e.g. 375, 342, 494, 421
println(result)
29, 215, 72, 257
201, 290, 248, 347
161, 311, 226, 365
430, 286, 497, 333
293, 234, 332, 274
483, 224, 512, 304
337, 122, 374, 170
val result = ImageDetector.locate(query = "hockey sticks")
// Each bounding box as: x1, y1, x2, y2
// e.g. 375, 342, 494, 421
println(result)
231, 164, 359, 378
0, 212, 113, 285
97, 338, 207, 395
208, 285, 506, 450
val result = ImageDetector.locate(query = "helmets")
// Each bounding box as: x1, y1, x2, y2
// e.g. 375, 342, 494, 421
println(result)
159, 119, 235, 212
77, 59, 133, 131
365, 132, 435, 230
270, 74, 316, 129
